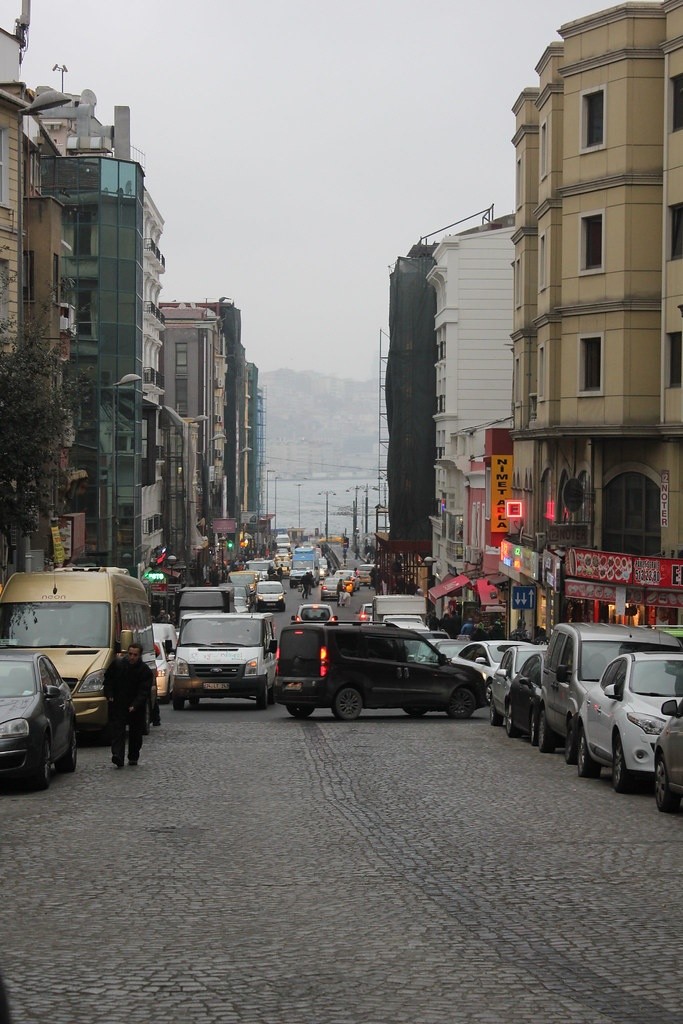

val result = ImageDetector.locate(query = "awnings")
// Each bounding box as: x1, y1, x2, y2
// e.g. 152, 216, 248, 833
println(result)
428, 575, 471, 605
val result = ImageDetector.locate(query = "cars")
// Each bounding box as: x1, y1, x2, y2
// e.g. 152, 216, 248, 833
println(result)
489, 644, 549, 725
246, 530, 361, 602
652, 696, 683, 812
505, 652, 569, 748
354, 594, 451, 661
0, 649, 78, 791
450, 640, 535, 706
426, 640, 475, 660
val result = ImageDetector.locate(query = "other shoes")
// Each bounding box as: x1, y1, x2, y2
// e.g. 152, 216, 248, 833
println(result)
128, 761, 137, 765
112, 754, 124, 767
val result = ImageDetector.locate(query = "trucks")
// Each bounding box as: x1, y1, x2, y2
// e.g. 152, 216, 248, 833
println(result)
171, 612, 279, 710
174, 586, 236, 630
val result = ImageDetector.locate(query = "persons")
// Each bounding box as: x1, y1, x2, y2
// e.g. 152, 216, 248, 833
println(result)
428, 611, 439, 631
368, 567, 383, 595
62, 606, 106, 642
365, 544, 373, 564
337, 579, 345, 607
156, 610, 170, 622
300, 570, 315, 600
211, 570, 220, 587
489, 623, 505, 640
102, 643, 154, 767
354, 568, 361, 590
450, 611, 460, 630
278, 563, 283, 584
343, 551, 347, 566
461, 617, 475, 634
267, 565, 275, 580
331, 567, 336, 575
470, 624, 490, 641
383, 575, 416, 595
439, 613, 456, 638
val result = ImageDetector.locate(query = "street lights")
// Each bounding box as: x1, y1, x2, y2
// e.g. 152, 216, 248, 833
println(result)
186, 414, 209, 584
209, 433, 226, 510
267, 469, 282, 529
318, 488, 337, 539
296, 483, 302, 528
166, 555, 177, 577
345, 483, 378, 536
112, 374, 143, 566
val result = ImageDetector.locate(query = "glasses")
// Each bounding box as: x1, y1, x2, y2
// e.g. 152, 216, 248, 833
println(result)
129, 652, 138, 656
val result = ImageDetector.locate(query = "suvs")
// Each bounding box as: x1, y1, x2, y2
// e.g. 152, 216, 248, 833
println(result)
576, 651, 683, 794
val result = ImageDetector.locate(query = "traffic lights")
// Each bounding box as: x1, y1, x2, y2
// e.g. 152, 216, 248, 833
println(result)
147, 570, 165, 580
227, 539, 233, 551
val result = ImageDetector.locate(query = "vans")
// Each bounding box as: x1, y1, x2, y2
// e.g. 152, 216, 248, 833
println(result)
538, 621, 683, 765
357, 563, 378, 586
254, 581, 288, 612
290, 602, 338, 625
154, 640, 176, 703
219, 582, 255, 613
275, 620, 488, 721
151, 623, 177, 654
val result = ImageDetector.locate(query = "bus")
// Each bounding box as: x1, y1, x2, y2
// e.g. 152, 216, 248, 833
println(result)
0, 566, 158, 737
